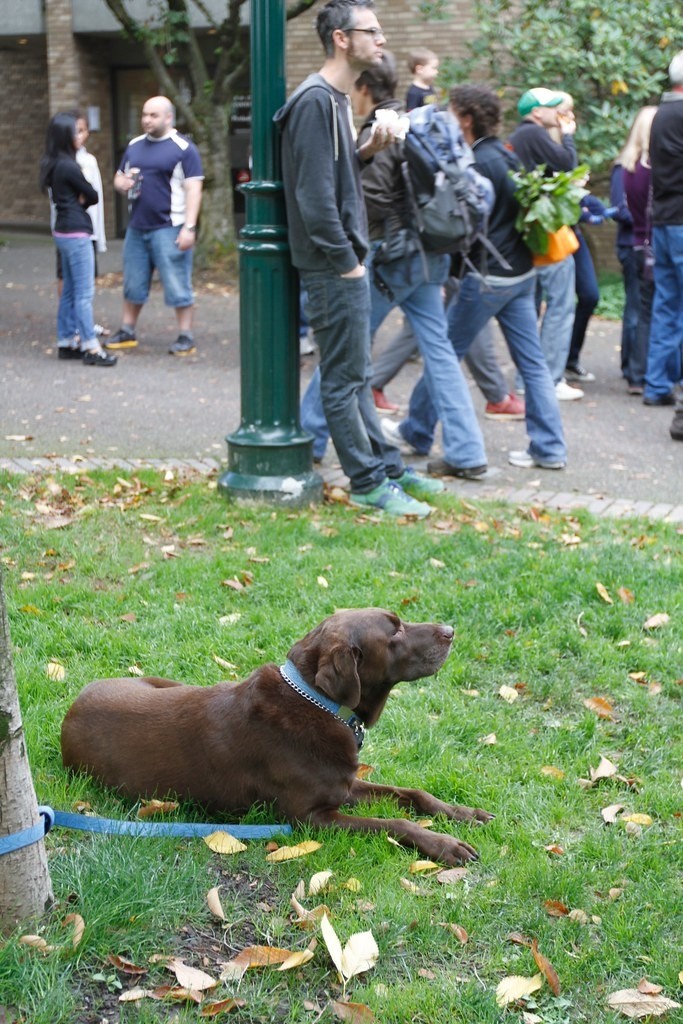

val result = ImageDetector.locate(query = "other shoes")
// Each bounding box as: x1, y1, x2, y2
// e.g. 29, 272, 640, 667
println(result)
670, 408, 683, 440
628, 385, 644, 395
644, 393, 676, 406
94, 324, 110, 336
84, 351, 117, 366
58, 346, 85, 359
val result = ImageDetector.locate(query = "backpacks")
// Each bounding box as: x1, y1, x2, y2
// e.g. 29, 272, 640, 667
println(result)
361, 103, 495, 255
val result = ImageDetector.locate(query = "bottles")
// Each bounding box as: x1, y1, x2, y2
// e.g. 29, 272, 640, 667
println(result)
127, 168, 142, 199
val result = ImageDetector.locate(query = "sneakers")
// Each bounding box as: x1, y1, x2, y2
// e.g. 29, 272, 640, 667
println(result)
169, 335, 197, 356
427, 459, 488, 479
350, 477, 431, 517
104, 329, 138, 348
508, 449, 566, 469
565, 363, 596, 381
372, 388, 399, 414
381, 418, 418, 457
515, 378, 583, 397
485, 392, 525, 420
390, 466, 444, 499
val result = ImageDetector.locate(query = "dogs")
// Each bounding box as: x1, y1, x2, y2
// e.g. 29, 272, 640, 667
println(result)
61, 607, 495, 867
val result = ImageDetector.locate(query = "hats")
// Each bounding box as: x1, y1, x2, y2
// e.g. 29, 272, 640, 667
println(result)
517, 88, 562, 116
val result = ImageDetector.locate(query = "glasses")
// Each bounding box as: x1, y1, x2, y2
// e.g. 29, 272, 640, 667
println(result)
345, 27, 378, 38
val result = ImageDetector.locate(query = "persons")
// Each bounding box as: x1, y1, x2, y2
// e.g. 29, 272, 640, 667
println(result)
299, 47, 683, 478
273, 0, 444, 521
102, 96, 204, 356
37, 105, 118, 367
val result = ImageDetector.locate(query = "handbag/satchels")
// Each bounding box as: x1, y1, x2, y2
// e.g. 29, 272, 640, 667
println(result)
643, 255, 656, 281
